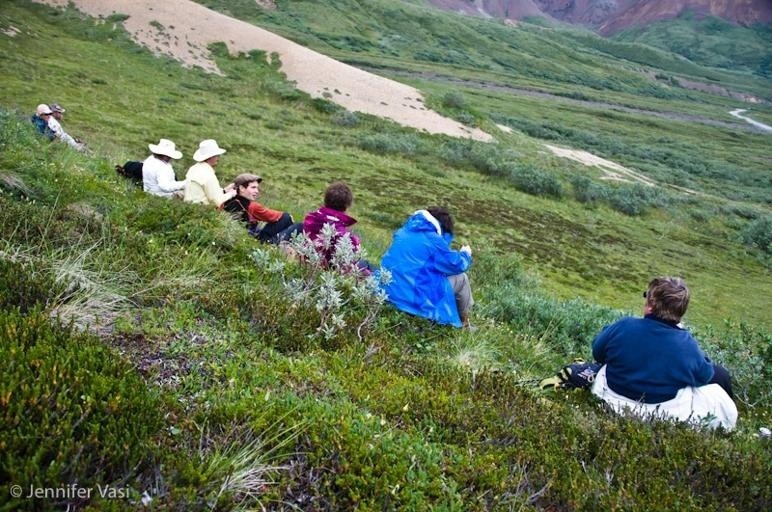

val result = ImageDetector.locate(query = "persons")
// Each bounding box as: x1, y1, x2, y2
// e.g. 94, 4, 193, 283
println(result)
183, 138, 237, 213
591, 275, 738, 434
370, 205, 476, 328
48, 102, 86, 152
304, 183, 381, 283
220, 174, 302, 246
142, 137, 186, 200
30, 103, 60, 145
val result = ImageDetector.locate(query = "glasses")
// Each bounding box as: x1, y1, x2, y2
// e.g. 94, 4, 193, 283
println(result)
644, 292, 646, 297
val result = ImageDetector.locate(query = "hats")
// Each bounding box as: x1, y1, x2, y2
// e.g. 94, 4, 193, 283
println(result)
192, 139, 227, 162
148, 138, 183, 160
35, 103, 66, 117
232, 174, 262, 190
